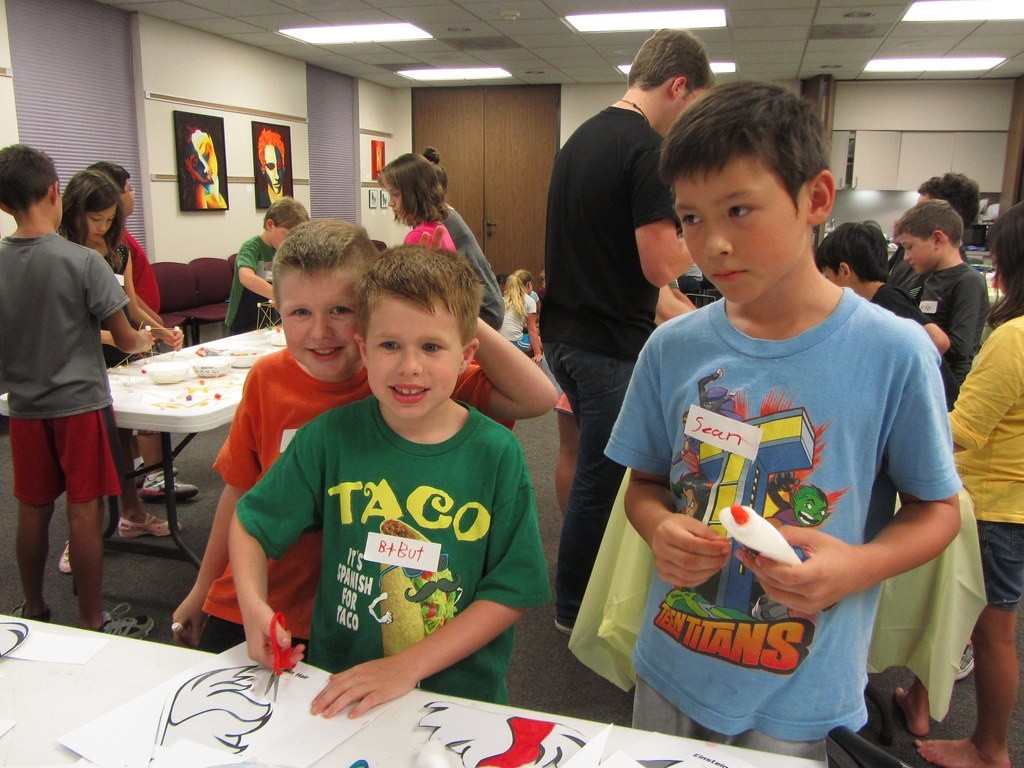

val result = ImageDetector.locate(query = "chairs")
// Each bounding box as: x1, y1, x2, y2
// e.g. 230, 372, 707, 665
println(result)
149, 252, 238, 347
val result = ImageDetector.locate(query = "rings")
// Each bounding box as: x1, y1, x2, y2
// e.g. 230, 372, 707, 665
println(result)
172, 622, 183, 632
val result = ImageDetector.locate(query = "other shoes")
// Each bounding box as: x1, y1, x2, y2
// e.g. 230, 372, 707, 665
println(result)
555, 616, 574, 636
956, 643, 976, 678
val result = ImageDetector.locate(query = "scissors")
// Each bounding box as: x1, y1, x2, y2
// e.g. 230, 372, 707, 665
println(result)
264, 612, 298, 702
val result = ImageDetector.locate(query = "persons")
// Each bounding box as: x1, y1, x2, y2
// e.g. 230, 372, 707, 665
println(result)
492, 265, 546, 362
887, 174, 979, 288
170, 218, 560, 654
226, 246, 554, 717
598, 80, 962, 758
542, 27, 714, 628
85, 159, 200, 500
57, 169, 183, 575
380, 143, 503, 331
888, 198, 988, 388
916, 198, 1024, 768
224, 194, 311, 339
811, 216, 962, 413
0, 142, 154, 636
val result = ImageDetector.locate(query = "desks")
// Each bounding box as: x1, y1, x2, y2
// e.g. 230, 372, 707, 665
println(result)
73, 325, 287, 595
0, 607, 826, 768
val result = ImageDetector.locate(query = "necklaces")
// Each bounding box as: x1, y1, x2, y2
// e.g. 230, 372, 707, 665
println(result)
618, 99, 650, 126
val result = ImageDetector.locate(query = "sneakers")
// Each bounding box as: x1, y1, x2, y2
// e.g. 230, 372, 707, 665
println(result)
12, 599, 51, 623
133, 464, 178, 487
99, 603, 153, 639
140, 471, 199, 499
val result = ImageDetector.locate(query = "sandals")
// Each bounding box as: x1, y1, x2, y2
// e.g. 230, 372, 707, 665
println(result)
58, 540, 75, 574
119, 514, 182, 537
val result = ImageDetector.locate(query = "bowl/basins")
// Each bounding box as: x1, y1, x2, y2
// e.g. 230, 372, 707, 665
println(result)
141, 362, 190, 384
191, 356, 231, 378
221, 348, 270, 368
